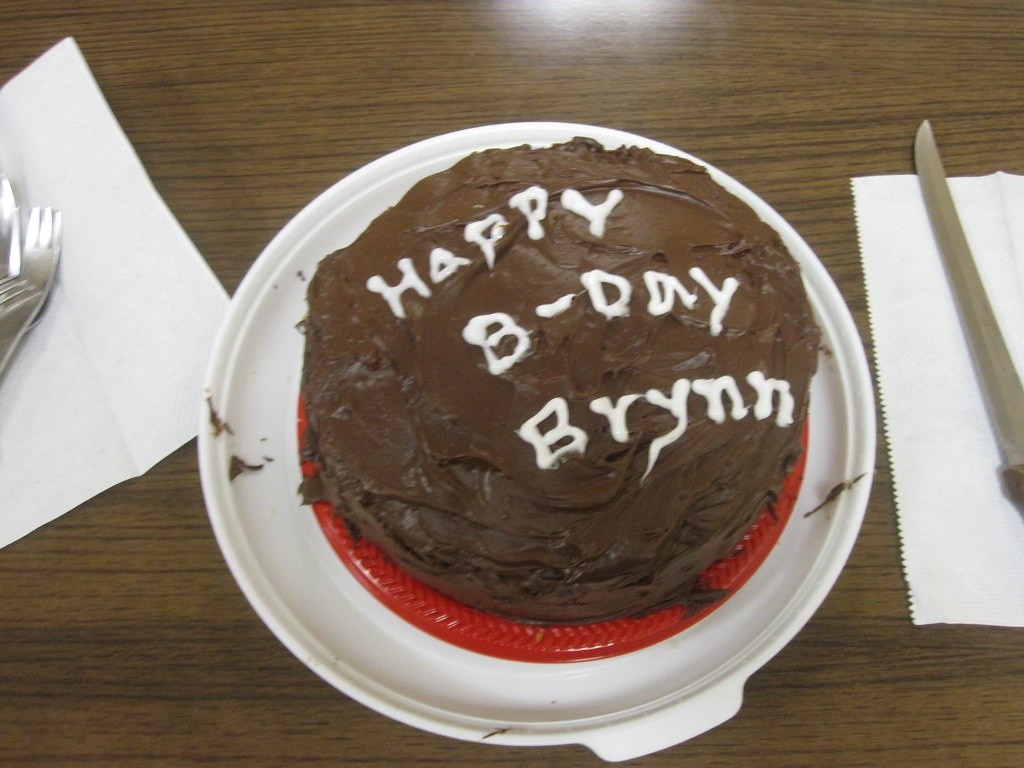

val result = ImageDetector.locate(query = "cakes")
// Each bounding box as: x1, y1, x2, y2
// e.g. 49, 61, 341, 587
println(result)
298, 133, 822, 626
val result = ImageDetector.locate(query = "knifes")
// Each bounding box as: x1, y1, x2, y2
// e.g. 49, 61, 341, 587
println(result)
914, 118, 1023, 517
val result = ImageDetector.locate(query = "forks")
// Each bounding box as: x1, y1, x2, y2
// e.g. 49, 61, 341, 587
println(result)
0, 274, 43, 318
0, 205, 63, 376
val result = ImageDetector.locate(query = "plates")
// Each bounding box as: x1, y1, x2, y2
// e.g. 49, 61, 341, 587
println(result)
294, 396, 809, 662
197, 120, 877, 761
